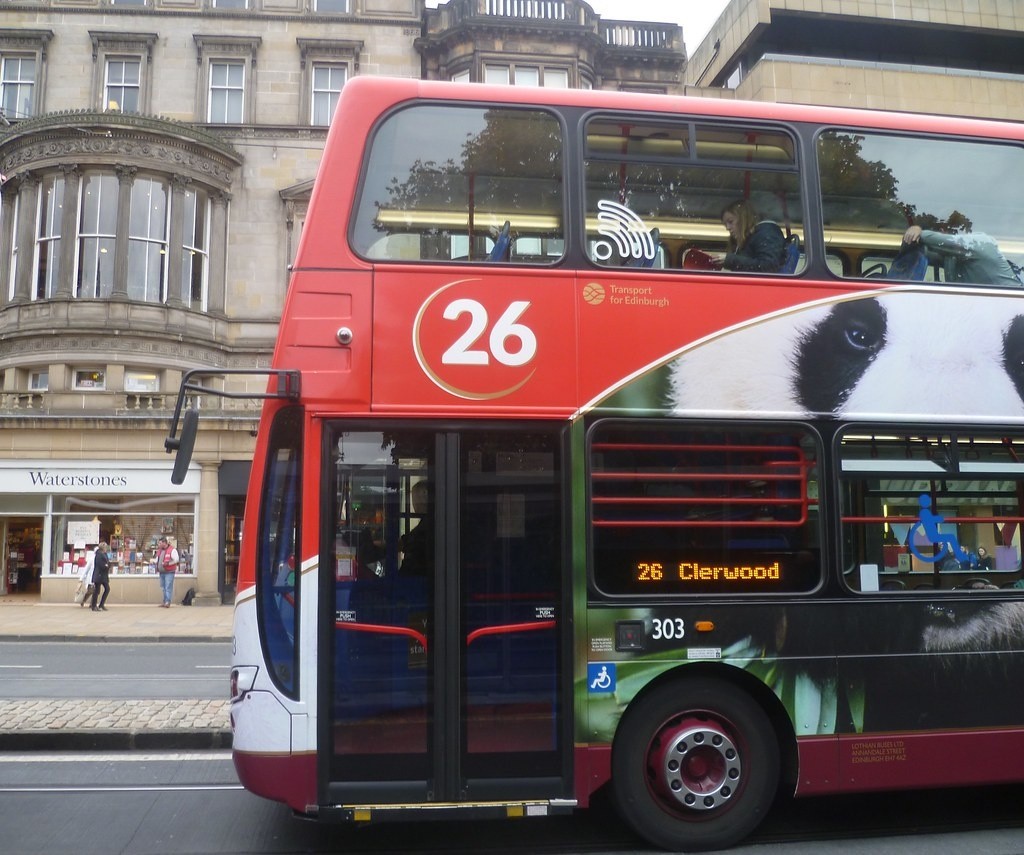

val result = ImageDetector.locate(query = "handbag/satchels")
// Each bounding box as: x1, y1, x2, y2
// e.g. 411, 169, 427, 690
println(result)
74, 582, 84, 603
88, 584, 96, 594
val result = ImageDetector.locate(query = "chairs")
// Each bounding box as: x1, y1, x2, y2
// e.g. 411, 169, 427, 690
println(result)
483, 220, 928, 282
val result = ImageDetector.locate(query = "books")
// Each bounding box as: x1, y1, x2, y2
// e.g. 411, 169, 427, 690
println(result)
57, 538, 158, 575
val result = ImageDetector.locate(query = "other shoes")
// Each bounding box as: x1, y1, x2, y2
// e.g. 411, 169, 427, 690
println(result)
81, 601, 85, 607
158, 601, 165, 607
92, 605, 101, 611
99, 604, 107, 610
165, 603, 170, 607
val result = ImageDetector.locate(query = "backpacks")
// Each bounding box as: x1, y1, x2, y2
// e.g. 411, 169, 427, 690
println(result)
181, 587, 195, 606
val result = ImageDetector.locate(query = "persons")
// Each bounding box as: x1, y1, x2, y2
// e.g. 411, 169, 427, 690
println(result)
708, 200, 786, 273
903, 207, 1023, 286
79, 547, 99, 607
976, 547, 992, 570
374, 479, 435, 613
960, 544, 977, 570
738, 453, 816, 593
942, 549, 961, 571
153, 538, 179, 608
92, 542, 111, 611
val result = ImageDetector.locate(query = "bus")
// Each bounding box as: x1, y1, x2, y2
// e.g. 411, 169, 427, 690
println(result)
163, 76, 1024, 855
